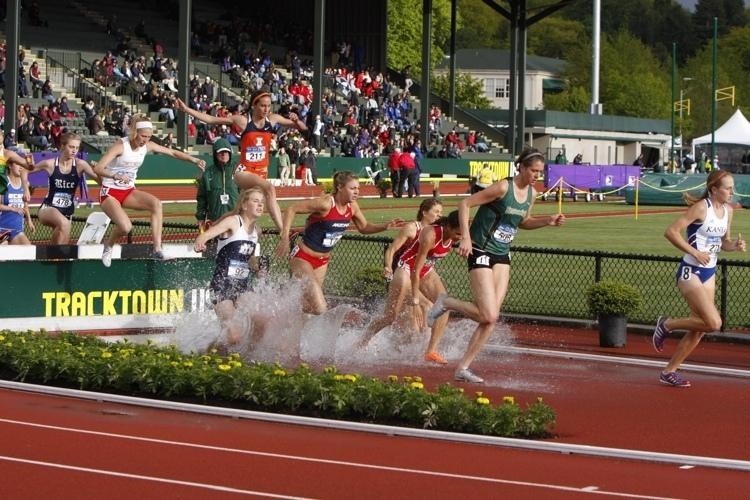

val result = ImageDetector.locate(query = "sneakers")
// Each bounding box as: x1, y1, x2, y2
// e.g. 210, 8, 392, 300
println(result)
421, 351, 449, 365
454, 367, 485, 385
652, 315, 673, 353
148, 247, 166, 259
278, 230, 300, 242
101, 243, 113, 269
425, 289, 451, 329
658, 370, 691, 388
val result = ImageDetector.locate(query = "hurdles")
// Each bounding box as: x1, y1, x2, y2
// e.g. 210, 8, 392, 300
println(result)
1, 239, 221, 338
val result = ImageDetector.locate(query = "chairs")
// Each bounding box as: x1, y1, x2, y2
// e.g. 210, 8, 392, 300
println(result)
435, 115, 521, 160
189, 62, 314, 156
324, 69, 421, 155
18, 48, 180, 154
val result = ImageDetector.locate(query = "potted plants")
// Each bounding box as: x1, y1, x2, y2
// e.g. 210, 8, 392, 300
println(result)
583, 276, 645, 351
374, 178, 392, 199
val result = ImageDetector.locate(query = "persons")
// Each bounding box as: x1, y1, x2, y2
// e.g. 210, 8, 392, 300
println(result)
633, 151, 750, 173
652, 170, 748, 388
